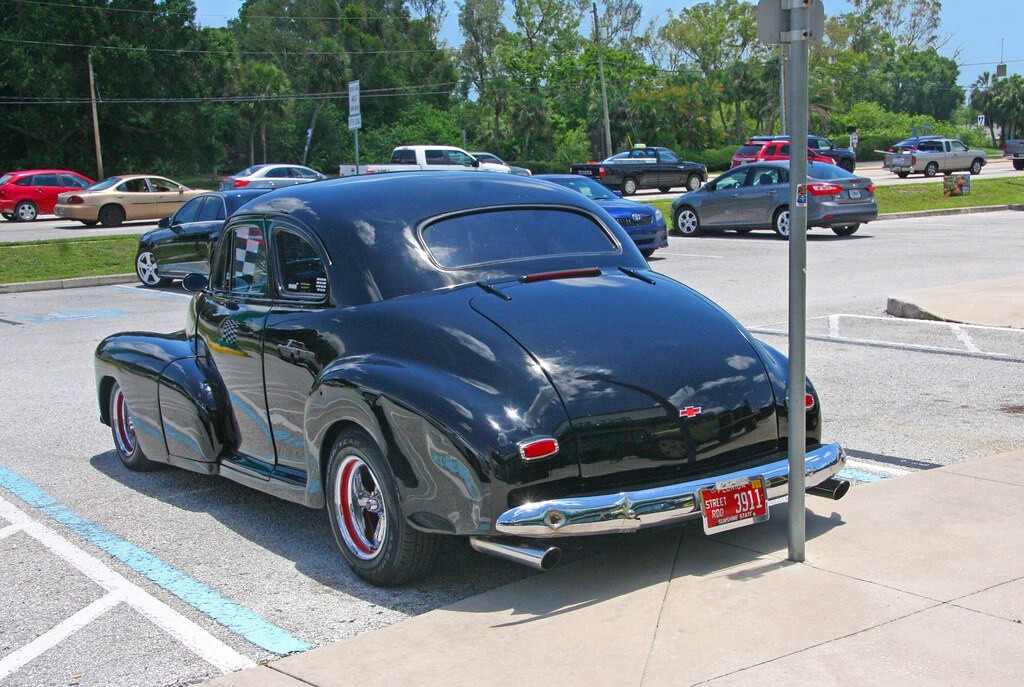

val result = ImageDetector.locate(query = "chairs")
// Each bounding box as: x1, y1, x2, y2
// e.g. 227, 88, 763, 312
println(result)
760, 174, 773, 185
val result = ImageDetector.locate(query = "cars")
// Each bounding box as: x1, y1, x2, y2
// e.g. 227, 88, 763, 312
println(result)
219, 163, 330, 191
888, 134, 963, 155
88, 167, 852, 588
670, 157, 878, 238
447, 150, 532, 177
0, 169, 97, 222
528, 173, 670, 258
134, 188, 277, 289
730, 140, 838, 169
53, 174, 216, 227
598, 151, 678, 162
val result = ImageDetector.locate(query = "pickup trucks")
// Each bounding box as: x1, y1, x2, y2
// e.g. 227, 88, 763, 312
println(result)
338, 144, 512, 177
881, 137, 988, 178
1002, 139, 1024, 170
569, 143, 708, 196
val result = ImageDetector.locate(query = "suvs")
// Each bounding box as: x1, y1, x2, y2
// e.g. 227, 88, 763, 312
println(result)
748, 133, 857, 173
